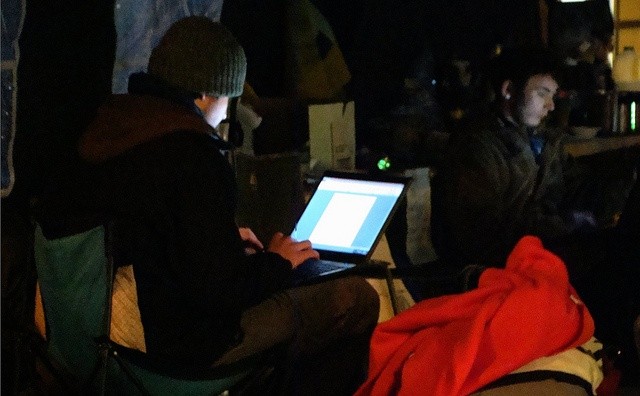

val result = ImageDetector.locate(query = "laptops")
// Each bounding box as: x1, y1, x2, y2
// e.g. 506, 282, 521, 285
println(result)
282, 169, 414, 291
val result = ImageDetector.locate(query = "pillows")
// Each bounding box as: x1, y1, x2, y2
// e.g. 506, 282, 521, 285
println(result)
32, 264, 149, 354
469, 334, 605, 396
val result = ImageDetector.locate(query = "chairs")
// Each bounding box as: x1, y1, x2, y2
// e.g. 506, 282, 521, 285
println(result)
32, 217, 288, 395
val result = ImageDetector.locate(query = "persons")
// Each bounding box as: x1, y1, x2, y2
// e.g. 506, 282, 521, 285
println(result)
63, 15, 380, 396
469, 54, 624, 247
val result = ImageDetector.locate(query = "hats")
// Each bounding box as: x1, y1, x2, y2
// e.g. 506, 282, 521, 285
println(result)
149, 15, 248, 97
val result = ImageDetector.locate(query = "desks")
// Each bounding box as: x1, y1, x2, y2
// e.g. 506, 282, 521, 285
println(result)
564, 134, 640, 158
340, 259, 400, 318
